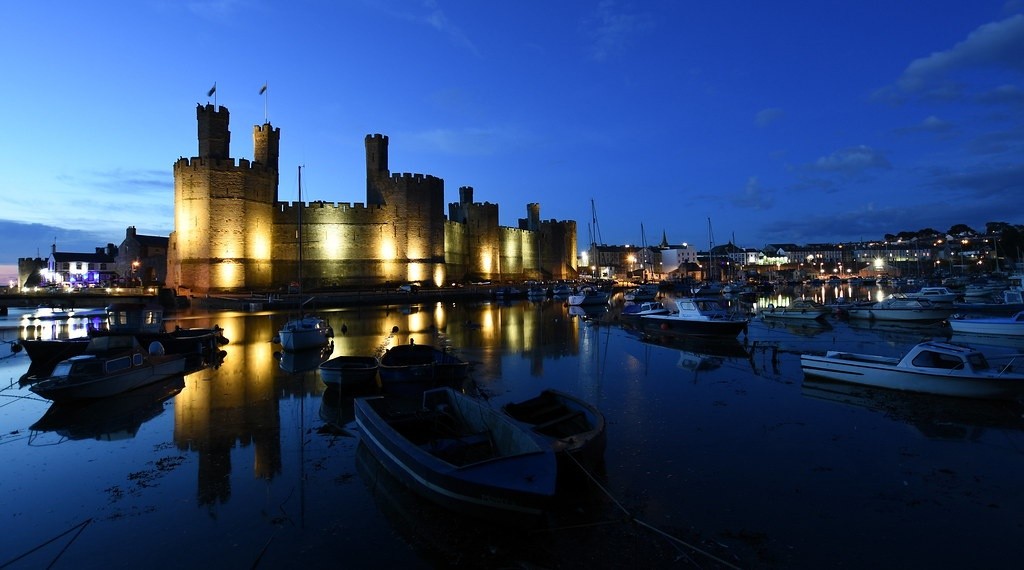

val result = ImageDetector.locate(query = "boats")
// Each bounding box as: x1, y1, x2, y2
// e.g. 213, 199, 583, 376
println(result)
553, 283, 574, 294
566, 286, 609, 306
379, 338, 469, 389
566, 306, 608, 326
953, 275, 1024, 306
762, 299, 833, 319
496, 285, 519, 299
823, 296, 878, 311
894, 287, 959, 302
947, 307, 1024, 336
621, 302, 671, 316
528, 283, 548, 296
318, 355, 379, 397
847, 295, 959, 320
991, 288, 1023, 305
641, 297, 749, 343
18, 302, 224, 363
800, 340, 1024, 400
354, 385, 559, 517
502, 388, 606, 459
960, 284, 995, 297
28, 338, 186, 403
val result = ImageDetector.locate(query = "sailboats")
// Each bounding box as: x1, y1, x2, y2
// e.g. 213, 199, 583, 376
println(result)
273, 165, 335, 360
691, 216, 721, 295
624, 222, 659, 302
723, 230, 736, 294
